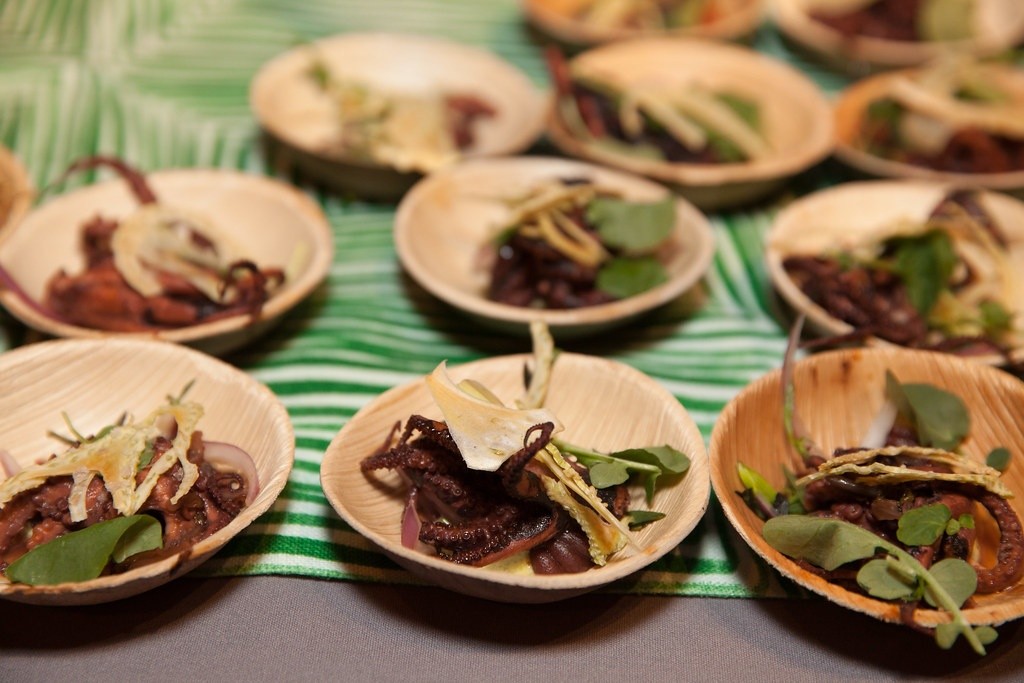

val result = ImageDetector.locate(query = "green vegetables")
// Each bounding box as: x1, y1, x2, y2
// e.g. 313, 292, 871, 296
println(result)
2, 0, 1004, 656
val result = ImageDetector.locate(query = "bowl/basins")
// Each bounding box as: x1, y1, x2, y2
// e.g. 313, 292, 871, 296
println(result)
543, 38, 837, 214
319, 351, 715, 600
393, 155, 717, 343
0, 142, 33, 255
0, 338, 293, 608
764, 179, 1024, 364
767, 0, 1024, 67
247, 32, 540, 199
707, 349, 1024, 627
519, 0, 767, 45
0, 172, 335, 356
830, 63, 1024, 192
